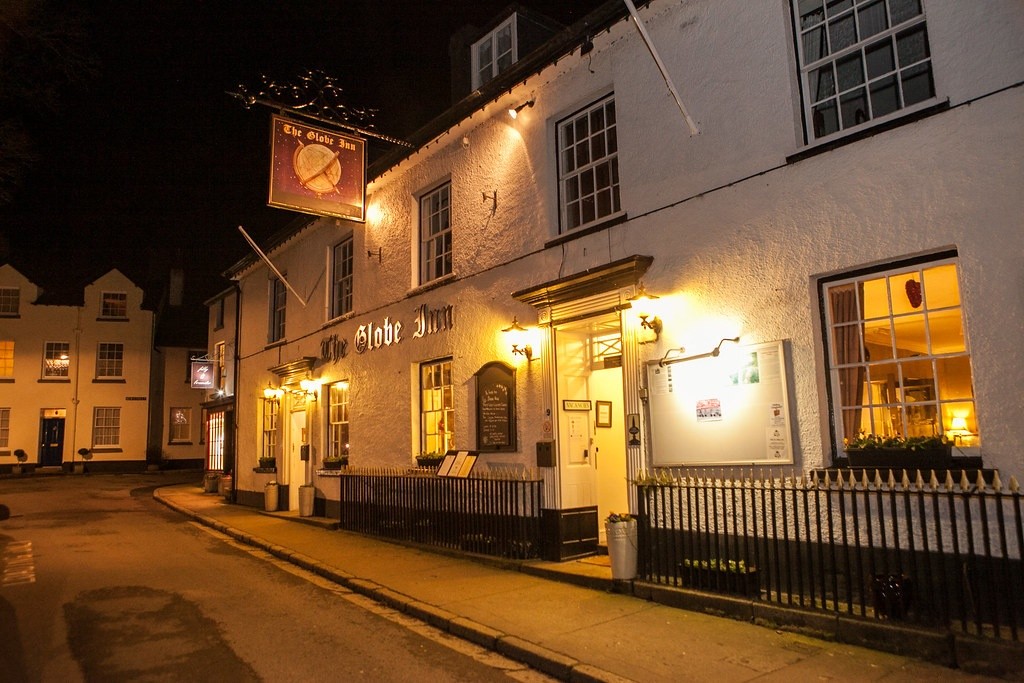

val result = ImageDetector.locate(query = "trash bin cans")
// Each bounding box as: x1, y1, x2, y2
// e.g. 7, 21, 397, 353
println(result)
203, 473, 219, 492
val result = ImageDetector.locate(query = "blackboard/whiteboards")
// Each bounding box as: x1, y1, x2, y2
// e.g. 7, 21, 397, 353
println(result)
641, 342, 799, 467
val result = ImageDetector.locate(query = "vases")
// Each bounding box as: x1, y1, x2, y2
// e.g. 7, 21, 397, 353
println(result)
300, 486, 316, 517
379, 527, 410, 540
843, 441, 957, 471
218, 480, 232, 495
510, 550, 530, 560
415, 523, 433, 544
461, 540, 496, 554
204, 480, 218, 493
605, 518, 638, 581
263, 483, 280, 512
322, 458, 348, 469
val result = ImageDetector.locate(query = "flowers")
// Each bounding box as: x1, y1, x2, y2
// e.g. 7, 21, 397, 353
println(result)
380, 520, 407, 526
417, 518, 429, 526
217, 474, 232, 479
200, 473, 218, 487
462, 532, 495, 543
326, 455, 341, 461
265, 481, 279, 486
603, 511, 632, 523
510, 537, 533, 553
844, 429, 949, 450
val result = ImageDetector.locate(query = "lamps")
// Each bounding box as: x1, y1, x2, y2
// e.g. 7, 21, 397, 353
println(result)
627, 282, 663, 335
658, 336, 740, 368
368, 247, 381, 263
503, 318, 536, 363
300, 371, 318, 402
482, 189, 497, 209
509, 101, 533, 119
265, 382, 282, 407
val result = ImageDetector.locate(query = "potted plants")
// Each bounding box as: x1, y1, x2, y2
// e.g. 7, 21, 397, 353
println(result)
12, 449, 25, 474
677, 558, 763, 600
416, 451, 444, 470
75, 448, 89, 473
147, 448, 160, 471
260, 457, 276, 468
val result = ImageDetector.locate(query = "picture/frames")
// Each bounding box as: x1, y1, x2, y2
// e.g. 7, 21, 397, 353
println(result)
596, 400, 613, 428
